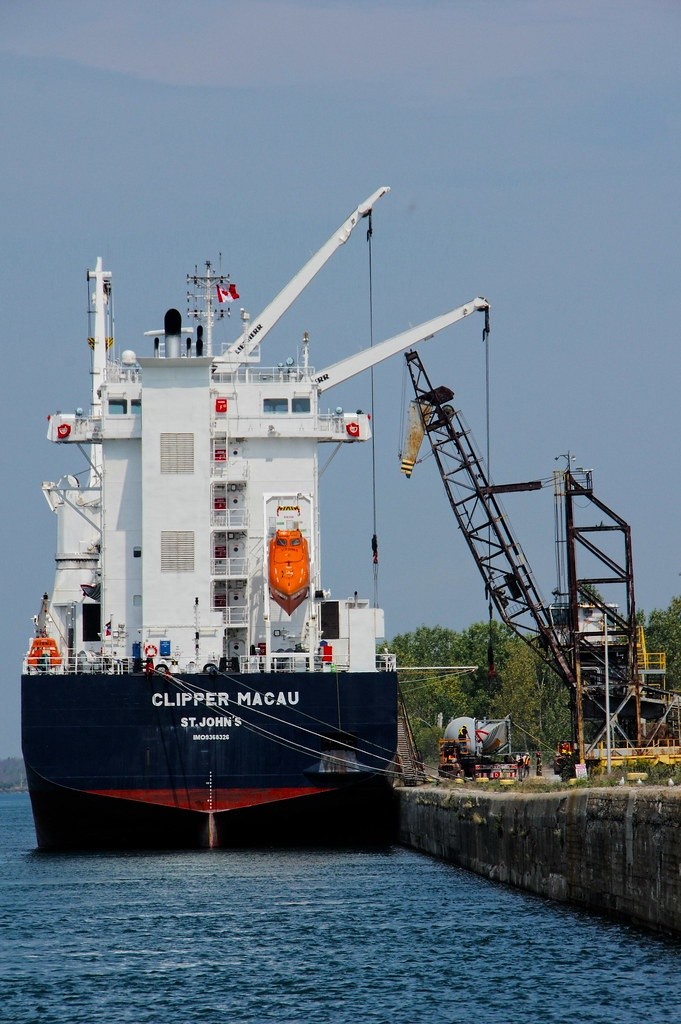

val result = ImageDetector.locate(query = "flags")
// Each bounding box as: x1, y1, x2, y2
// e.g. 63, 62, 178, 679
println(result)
106, 622, 111, 636
216, 283, 239, 303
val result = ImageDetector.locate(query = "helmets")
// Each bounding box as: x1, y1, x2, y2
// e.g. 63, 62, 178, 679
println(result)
536, 752, 541, 756
526, 752, 529, 756
463, 724, 467, 728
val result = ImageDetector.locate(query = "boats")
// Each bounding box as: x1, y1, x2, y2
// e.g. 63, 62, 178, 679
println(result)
21, 184, 490, 854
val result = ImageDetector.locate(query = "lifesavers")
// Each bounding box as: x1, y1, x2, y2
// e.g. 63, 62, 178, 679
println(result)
145, 644, 158, 658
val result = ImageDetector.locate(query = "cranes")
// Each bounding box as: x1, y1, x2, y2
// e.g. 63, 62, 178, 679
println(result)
397, 349, 666, 724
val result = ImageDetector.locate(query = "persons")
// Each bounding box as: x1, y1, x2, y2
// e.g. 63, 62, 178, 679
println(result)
536, 753, 542, 775
517, 754, 523, 782
557, 742, 579, 782
523, 753, 530, 777
458, 724, 470, 752
